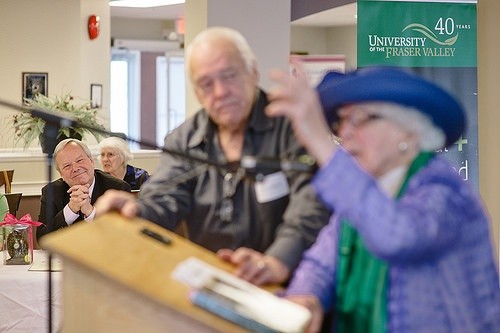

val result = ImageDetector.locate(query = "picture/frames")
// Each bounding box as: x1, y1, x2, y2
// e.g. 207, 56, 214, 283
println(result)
91, 85, 103, 108
23, 72, 48, 107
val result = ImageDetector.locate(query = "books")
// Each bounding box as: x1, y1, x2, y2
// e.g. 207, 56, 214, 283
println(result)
193, 275, 311, 332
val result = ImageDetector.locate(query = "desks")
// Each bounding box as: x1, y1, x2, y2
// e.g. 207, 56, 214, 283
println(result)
0, 250, 63, 333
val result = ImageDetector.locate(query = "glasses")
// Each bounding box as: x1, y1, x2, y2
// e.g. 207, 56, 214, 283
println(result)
331, 110, 383, 132
219, 173, 237, 223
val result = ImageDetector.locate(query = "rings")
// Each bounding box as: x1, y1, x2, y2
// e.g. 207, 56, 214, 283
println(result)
258, 262, 267, 268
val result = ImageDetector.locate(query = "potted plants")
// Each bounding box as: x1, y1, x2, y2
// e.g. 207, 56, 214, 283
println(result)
4, 94, 107, 153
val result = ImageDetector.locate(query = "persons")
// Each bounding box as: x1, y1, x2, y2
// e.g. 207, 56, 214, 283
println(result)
98, 136, 150, 190
264, 56, 500, 333
36, 138, 131, 249
95, 27, 333, 285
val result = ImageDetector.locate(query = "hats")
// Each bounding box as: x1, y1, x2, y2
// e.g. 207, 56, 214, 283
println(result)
316, 66, 466, 151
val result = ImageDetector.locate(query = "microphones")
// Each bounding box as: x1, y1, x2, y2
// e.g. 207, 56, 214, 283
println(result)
243, 155, 317, 176
